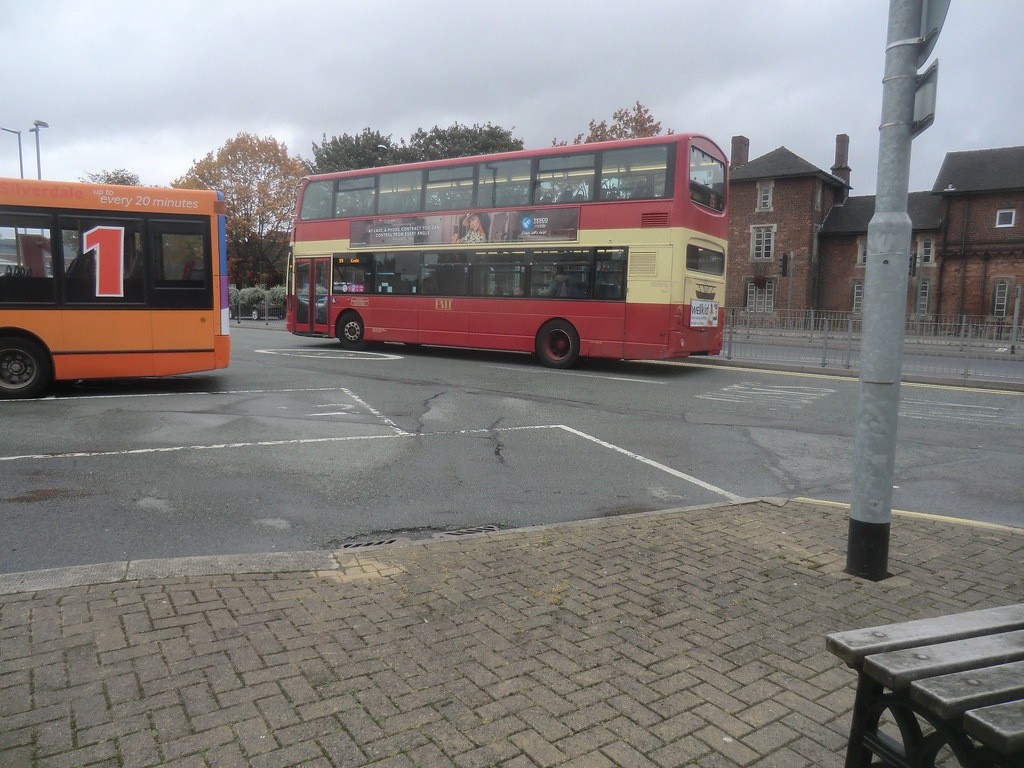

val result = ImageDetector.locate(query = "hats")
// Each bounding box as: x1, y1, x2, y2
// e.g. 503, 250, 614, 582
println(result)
463, 213, 473, 226
554, 266, 566, 275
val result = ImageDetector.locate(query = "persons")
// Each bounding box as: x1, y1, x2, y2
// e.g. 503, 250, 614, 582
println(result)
546, 264, 571, 298
452, 213, 490, 243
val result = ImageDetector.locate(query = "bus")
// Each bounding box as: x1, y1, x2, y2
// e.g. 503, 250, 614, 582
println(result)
0, 175, 230, 398
284, 131, 732, 370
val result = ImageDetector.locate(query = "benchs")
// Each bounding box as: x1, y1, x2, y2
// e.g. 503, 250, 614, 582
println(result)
826, 603, 1024, 768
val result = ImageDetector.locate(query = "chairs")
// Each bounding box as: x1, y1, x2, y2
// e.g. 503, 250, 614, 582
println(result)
5, 265, 31, 277
598, 280, 622, 298
335, 182, 644, 217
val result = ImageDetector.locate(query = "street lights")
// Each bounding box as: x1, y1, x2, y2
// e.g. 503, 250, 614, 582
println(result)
31, 121, 52, 178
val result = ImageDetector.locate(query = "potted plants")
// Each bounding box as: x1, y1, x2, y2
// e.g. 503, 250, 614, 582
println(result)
754, 275, 766, 289
